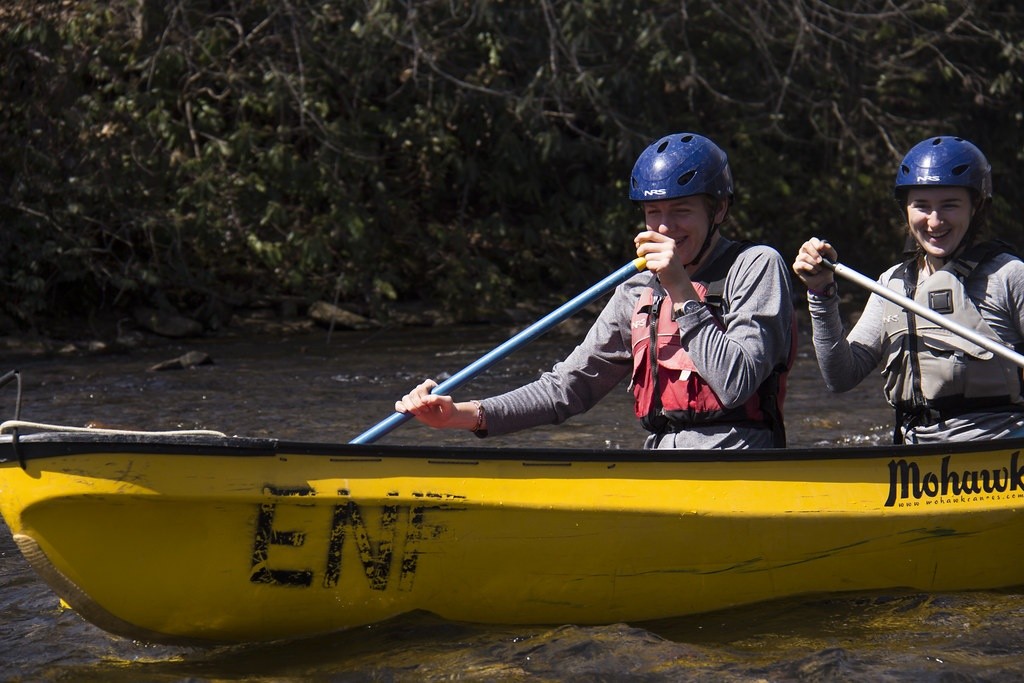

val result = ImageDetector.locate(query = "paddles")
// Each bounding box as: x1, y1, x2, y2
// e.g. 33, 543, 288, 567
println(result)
346, 256, 648, 446
798, 250, 1024, 366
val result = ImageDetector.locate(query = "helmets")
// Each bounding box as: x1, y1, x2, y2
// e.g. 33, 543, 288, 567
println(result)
894, 136, 993, 207
628, 132, 735, 207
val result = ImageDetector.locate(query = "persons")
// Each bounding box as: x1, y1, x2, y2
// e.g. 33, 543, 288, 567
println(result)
793, 135, 1024, 445
395, 133, 797, 449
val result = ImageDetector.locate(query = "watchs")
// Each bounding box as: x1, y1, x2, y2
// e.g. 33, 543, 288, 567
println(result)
675, 299, 704, 319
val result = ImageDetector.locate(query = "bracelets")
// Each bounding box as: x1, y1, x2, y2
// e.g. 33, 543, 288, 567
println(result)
469, 400, 485, 433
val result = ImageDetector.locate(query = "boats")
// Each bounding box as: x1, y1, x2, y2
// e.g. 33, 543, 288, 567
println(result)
0, 367, 1024, 651
